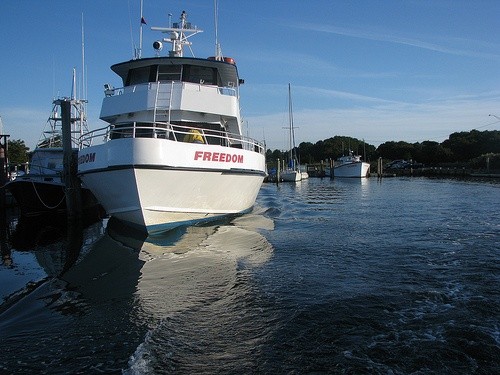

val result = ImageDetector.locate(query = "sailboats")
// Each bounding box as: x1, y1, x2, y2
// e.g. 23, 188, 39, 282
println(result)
279, 81, 303, 183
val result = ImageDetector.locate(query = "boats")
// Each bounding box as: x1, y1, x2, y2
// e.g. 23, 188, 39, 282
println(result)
0, 65, 107, 232
78, 0, 269, 236
326, 147, 371, 177
